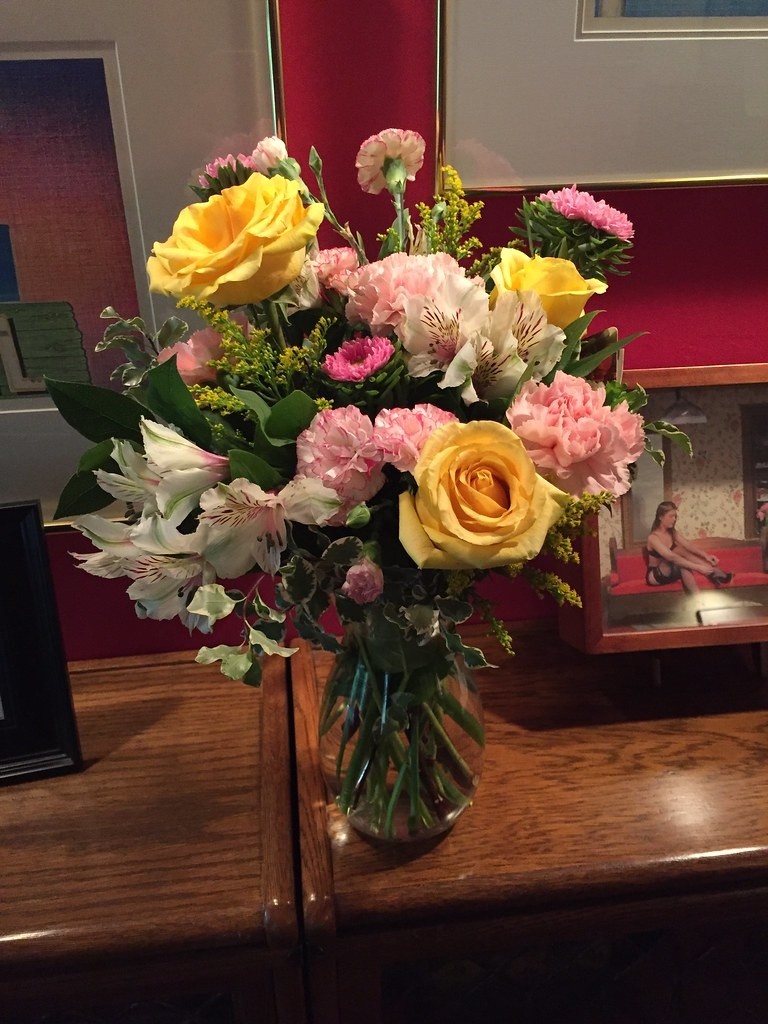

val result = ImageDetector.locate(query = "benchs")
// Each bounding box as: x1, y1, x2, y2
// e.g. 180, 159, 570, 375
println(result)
604, 536, 768, 628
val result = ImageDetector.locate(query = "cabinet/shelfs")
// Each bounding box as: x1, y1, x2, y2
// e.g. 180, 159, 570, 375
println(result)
0, 642, 312, 1024
289, 614, 768, 1024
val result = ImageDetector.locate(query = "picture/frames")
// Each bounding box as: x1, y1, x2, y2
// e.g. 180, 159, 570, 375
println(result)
0, 499, 82, 784
434, 0, 768, 205
560, 364, 768, 653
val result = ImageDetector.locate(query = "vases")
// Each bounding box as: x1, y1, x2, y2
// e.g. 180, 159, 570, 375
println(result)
319, 594, 487, 846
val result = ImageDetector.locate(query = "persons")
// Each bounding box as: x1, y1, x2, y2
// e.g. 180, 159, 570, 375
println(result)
646, 501, 736, 596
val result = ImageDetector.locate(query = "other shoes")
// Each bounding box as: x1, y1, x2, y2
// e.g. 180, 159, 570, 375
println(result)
708, 572, 736, 585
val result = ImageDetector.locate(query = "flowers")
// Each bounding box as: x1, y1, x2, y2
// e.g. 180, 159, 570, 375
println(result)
41, 128, 696, 842
756, 503, 768, 535
